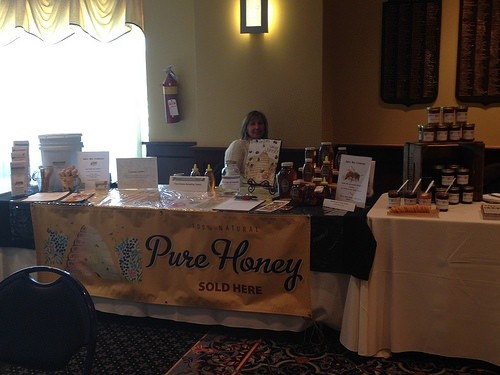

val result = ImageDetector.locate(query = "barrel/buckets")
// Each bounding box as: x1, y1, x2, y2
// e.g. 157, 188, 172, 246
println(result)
39, 134, 83, 169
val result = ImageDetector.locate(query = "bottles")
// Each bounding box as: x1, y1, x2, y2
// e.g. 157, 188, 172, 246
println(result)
291, 180, 305, 203
190, 164, 200, 177
304, 184, 316, 205
336, 147, 347, 171
313, 178, 322, 186
299, 167, 338, 200
278, 162, 297, 196
317, 177, 330, 209
303, 159, 314, 183
319, 143, 333, 169
305, 147, 316, 168
321, 156, 332, 184
205, 165, 214, 194
222, 161, 241, 193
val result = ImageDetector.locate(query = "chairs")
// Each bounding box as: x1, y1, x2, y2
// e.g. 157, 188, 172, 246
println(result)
0, 266, 97, 375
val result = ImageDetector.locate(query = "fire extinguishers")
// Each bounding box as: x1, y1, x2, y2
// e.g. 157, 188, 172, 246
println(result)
162, 64, 183, 123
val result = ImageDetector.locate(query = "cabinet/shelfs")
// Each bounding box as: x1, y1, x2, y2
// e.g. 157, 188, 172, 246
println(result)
403, 142, 485, 202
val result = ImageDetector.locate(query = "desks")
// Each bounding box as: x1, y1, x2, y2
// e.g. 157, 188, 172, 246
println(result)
29, 189, 377, 332
0, 192, 35, 249
339, 192, 499, 368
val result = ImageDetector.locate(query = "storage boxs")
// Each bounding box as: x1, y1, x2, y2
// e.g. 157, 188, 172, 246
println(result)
39, 134, 84, 188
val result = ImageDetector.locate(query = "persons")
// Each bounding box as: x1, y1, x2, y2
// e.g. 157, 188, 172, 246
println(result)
219, 111, 281, 189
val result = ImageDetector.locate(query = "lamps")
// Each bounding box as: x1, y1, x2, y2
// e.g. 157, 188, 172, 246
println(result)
240, 0, 269, 34
